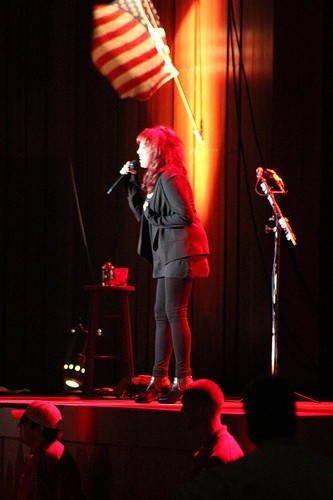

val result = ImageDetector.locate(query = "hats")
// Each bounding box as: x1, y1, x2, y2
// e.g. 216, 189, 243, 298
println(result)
182, 378, 225, 410
25, 399, 63, 430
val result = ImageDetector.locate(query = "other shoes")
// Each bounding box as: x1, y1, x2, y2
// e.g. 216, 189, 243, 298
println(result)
134, 375, 172, 403
158, 376, 194, 404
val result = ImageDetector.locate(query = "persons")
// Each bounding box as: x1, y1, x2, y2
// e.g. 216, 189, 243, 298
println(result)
120, 125, 211, 404
0, 379, 333, 500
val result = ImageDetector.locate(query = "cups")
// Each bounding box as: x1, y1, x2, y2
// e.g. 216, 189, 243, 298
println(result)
115, 266, 129, 287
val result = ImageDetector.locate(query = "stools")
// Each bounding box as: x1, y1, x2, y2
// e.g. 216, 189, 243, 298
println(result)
84, 284, 136, 396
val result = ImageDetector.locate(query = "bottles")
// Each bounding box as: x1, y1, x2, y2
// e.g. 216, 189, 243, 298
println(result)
101, 262, 116, 286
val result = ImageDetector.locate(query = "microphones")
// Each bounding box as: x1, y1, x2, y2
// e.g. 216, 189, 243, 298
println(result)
106, 160, 138, 194
